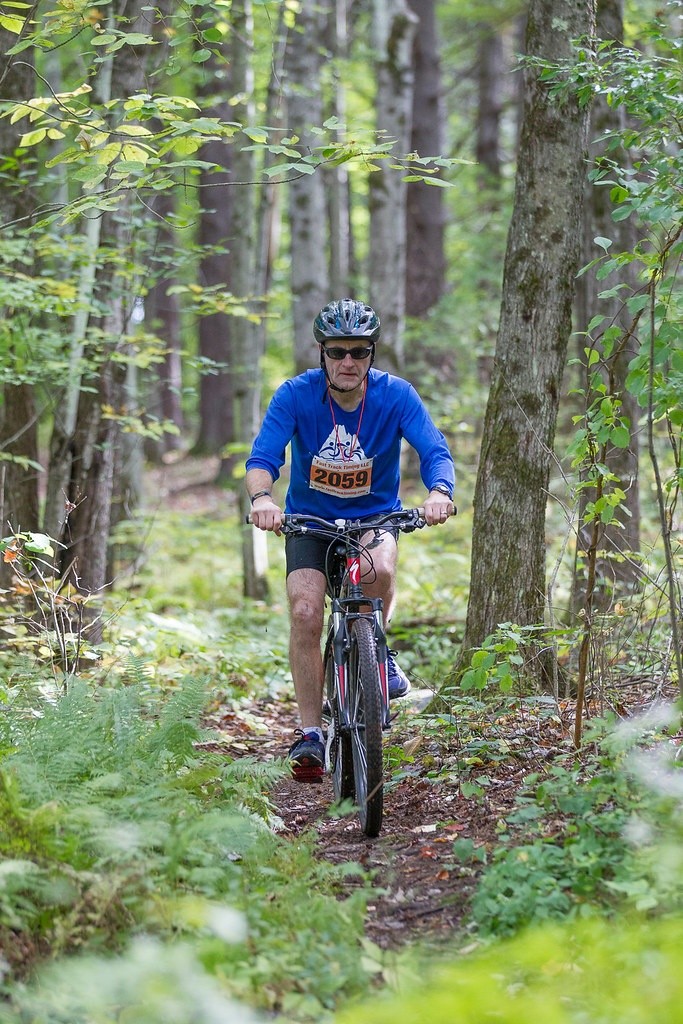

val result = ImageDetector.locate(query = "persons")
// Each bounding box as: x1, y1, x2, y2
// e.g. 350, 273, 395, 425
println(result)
245, 298, 456, 782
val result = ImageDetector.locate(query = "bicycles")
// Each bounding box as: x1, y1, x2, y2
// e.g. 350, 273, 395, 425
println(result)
243, 503, 461, 840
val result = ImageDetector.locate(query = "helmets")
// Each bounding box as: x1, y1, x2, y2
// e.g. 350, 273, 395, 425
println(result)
313, 298, 381, 343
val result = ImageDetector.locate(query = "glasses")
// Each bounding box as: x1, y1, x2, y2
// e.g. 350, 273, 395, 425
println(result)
320, 344, 374, 360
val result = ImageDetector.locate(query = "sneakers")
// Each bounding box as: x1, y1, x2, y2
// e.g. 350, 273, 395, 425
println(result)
287, 728, 325, 784
384, 646, 412, 698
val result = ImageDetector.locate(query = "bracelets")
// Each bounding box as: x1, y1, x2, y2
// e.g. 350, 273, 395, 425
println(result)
251, 490, 272, 505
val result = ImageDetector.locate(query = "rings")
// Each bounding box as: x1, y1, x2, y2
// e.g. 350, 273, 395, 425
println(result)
441, 511, 447, 514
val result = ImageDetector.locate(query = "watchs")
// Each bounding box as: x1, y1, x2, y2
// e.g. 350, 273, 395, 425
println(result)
432, 485, 450, 495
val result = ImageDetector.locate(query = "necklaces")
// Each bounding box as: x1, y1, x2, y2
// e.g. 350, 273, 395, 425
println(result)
327, 376, 367, 464
